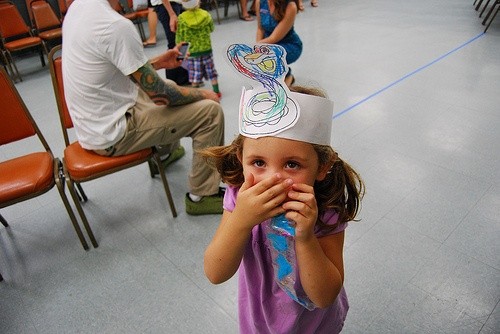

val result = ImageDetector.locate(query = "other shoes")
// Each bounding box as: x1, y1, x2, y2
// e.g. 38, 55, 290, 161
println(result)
185, 187, 226, 216
148, 145, 185, 175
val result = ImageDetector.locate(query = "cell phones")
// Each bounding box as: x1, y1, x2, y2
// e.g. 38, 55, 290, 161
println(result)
176, 42, 189, 60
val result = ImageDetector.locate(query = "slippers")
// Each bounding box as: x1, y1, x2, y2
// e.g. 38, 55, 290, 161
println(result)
239, 14, 254, 21
143, 40, 156, 48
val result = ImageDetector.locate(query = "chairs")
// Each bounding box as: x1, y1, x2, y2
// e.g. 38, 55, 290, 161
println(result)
48, 45, 178, 248
0, 67, 89, 282
1, 0, 241, 84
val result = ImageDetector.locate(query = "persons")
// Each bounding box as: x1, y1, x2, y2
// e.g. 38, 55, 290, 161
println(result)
142, 0, 164, 46
298, 0, 319, 11
197, 86, 365, 334
62, 0, 226, 216
255, 0, 303, 88
240, 0, 257, 21
151, 0, 205, 87
175, 0, 222, 99
